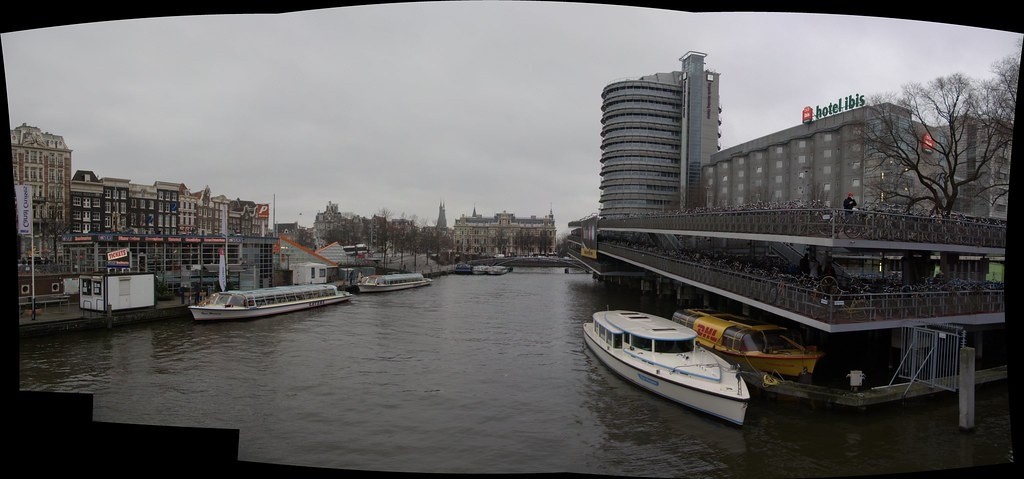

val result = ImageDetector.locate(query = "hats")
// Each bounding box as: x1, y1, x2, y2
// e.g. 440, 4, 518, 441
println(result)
848, 192, 853, 196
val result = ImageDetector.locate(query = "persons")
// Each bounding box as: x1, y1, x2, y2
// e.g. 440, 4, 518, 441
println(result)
844, 192, 857, 218
350, 270, 364, 284
800, 250, 831, 277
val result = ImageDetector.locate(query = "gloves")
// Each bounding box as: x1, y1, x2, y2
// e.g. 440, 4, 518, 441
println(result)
849, 201, 853, 204
851, 197, 854, 200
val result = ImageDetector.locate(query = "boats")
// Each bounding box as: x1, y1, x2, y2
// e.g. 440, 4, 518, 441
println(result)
486, 265, 509, 276
673, 307, 822, 375
582, 309, 750, 426
472, 265, 488, 275
188, 283, 351, 321
357, 272, 432, 293
454, 263, 472, 274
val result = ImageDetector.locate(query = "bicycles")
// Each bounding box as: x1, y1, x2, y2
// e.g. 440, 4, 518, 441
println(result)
862, 202, 1006, 247
723, 199, 862, 239
834, 277, 1004, 319
597, 235, 644, 263
601, 206, 723, 230
644, 245, 717, 284
718, 258, 838, 321
